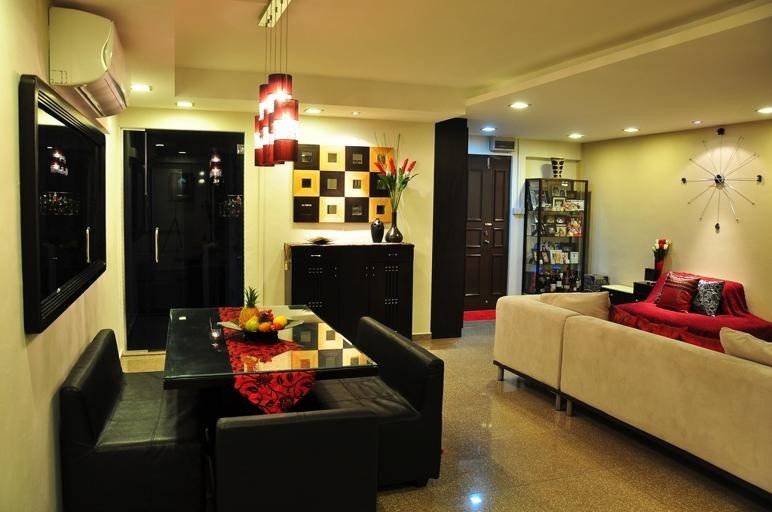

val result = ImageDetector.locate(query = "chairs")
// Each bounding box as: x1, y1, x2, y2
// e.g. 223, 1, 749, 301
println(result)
215, 405, 380, 512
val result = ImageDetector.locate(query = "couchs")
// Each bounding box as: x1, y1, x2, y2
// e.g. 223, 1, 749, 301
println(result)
491, 290, 772, 495
611, 270, 772, 353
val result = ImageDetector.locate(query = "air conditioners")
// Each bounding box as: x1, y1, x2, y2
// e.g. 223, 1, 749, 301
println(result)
48, 5, 130, 120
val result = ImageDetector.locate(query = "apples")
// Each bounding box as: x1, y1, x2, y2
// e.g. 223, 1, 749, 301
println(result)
258, 315, 287, 333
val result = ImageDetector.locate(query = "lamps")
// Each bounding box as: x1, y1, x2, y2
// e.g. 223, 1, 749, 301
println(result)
253, 0, 299, 167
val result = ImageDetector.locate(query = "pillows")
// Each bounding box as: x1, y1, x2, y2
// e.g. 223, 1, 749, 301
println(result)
720, 326, 772, 368
652, 272, 726, 318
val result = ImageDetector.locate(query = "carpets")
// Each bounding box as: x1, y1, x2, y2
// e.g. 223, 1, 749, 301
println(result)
463, 309, 496, 321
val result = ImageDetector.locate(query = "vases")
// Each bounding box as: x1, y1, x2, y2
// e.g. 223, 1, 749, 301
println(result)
371, 217, 384, 243
550, 157, 565, 179
385, 212, 403, 243
654, 257, 665, 271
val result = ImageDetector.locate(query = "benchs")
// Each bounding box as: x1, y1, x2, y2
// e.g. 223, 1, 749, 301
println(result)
59, 328, 200, 512
313, 315, 445, 493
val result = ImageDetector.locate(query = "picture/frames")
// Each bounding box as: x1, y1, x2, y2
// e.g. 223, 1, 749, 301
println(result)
531, 184, 585, 265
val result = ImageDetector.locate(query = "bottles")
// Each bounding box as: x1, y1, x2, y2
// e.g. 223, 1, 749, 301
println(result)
539, 261, 582, 292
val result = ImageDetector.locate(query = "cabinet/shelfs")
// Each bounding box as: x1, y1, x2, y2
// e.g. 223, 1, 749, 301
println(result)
521, 177, 589, 296
283, 241, 415, 342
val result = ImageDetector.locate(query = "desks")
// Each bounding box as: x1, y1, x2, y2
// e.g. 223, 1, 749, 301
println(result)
601, 281, 656, 305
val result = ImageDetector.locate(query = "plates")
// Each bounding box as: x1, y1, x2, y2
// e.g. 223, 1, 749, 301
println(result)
216, 317, 305, 337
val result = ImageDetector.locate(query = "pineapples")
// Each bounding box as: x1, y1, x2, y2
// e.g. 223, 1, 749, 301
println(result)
238, 285, 259, 330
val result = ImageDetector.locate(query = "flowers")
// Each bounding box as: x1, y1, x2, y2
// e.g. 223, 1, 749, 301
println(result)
652, 238, 673, 264
372, 130, 420, 214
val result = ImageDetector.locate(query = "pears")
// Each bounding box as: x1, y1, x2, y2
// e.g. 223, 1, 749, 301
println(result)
245, 315, 258, 332
